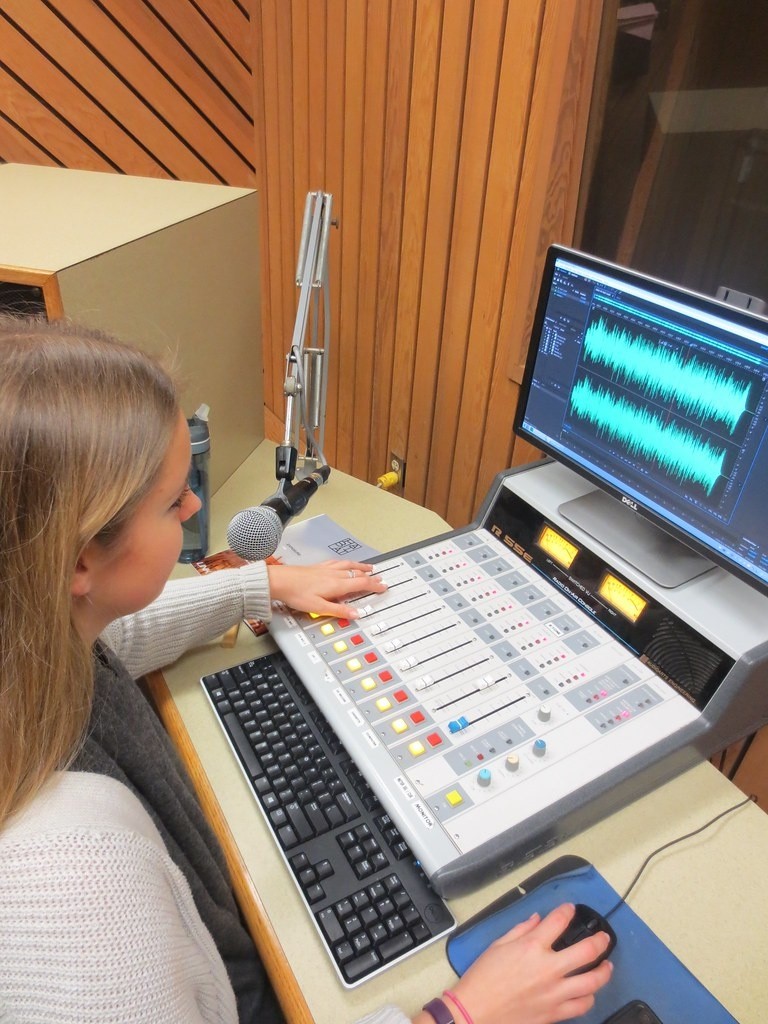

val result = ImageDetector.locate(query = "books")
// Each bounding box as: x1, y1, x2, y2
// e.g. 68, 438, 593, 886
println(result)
190, 514, 382, 638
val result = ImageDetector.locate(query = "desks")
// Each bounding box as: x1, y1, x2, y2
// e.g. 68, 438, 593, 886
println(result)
0, 162, 768, 1024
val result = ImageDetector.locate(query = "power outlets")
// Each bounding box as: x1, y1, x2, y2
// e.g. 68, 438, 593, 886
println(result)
390, 450, 405, 494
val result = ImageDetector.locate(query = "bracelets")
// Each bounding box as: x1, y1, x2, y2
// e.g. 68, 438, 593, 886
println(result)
422, 998, 456, 1024
443, 991, 474, 1024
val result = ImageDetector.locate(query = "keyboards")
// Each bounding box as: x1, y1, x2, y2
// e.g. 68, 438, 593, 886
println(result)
201, 648, 458, 990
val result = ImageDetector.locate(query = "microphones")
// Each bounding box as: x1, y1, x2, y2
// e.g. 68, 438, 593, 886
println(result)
227, 465, 332, 561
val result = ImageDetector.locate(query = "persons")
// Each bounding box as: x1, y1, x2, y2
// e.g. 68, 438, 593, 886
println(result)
0, 311, 615, 1024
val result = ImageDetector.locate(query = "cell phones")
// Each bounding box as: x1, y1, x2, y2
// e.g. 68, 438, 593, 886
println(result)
601, 1000, 663, 1024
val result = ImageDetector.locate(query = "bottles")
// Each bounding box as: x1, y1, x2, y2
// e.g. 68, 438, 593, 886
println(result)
173, 403, 210, 564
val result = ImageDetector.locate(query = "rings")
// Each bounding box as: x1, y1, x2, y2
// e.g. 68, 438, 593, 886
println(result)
348, 569, 356, 578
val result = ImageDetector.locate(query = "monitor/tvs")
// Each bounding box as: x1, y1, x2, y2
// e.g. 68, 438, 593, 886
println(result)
513, 244, 768, 596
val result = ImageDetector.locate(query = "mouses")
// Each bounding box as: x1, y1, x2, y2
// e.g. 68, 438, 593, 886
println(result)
552, 904, 618, 977
